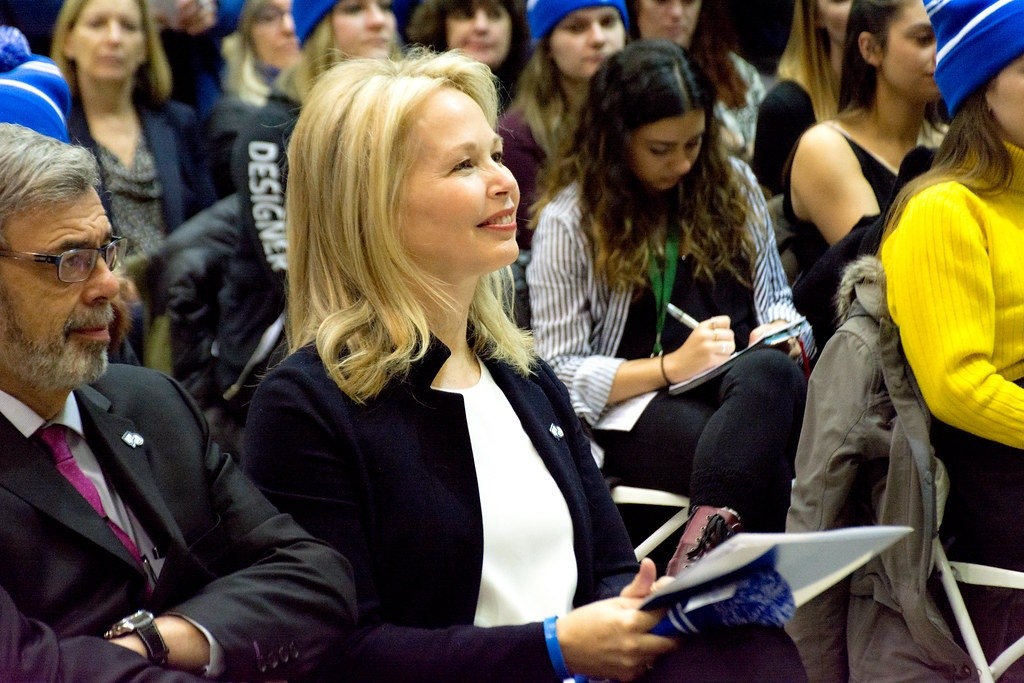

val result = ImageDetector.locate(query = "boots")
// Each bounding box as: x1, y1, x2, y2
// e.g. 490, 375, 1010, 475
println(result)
667, 504, 744, 579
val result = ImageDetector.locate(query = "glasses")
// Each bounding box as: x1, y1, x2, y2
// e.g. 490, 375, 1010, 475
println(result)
1, 236, 128, 283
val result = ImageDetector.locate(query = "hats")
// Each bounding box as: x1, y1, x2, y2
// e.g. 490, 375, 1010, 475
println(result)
525, 0, 628, 53
0, 25, 73, 145
290, 0, 407, 45
922, 0, 1024, 119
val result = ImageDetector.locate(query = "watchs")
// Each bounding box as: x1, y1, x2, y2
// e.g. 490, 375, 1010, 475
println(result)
102, 610, 169, 668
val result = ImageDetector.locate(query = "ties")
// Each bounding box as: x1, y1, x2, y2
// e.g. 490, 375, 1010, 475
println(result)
37, 424, 148, 584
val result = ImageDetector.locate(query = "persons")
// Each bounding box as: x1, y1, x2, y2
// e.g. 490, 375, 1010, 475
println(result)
0, 0, 1024, 582
241, 41, 811, 683
0, 121, 358, 683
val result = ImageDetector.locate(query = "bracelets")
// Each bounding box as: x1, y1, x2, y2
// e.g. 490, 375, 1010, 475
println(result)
543, 616, 570, 679
658, 351, 671, 391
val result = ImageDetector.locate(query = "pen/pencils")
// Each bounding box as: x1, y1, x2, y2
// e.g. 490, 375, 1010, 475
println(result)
667, 303, 700, 330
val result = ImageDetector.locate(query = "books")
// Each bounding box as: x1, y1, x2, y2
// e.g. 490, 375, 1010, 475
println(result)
666, 315, 802, 396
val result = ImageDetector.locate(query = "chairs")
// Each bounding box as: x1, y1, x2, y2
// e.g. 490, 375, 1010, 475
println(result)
782, 254, 1023, 682
